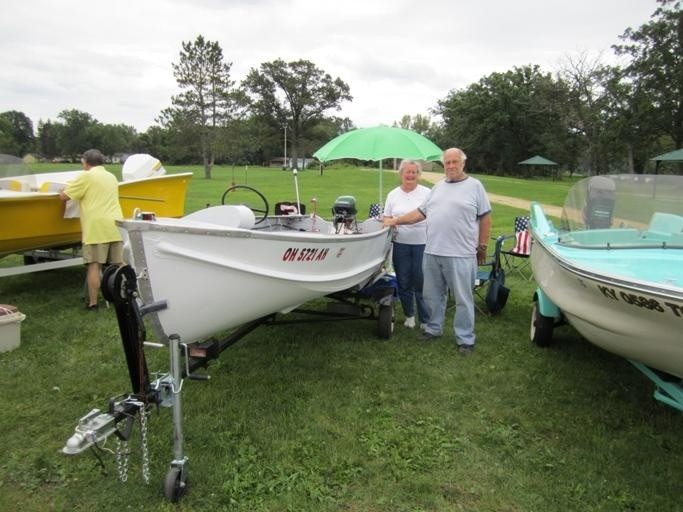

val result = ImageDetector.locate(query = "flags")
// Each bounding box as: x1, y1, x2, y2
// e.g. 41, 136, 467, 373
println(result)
514, 217, 539, 258
366, 201, 385, 221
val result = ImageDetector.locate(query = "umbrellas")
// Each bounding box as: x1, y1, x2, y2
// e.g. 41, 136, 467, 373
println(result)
312, 123, 443, 201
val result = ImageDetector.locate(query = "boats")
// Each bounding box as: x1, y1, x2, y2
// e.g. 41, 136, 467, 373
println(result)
1, 171, 193, 278
530, 175, 683, 410
63, 204, 396, 500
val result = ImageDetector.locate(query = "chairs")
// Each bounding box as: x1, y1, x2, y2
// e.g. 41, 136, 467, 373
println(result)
446, 234, 504, 317
501, 216, 534, 281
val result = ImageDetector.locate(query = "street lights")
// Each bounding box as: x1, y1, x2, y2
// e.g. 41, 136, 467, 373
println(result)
282, 123, 288, 165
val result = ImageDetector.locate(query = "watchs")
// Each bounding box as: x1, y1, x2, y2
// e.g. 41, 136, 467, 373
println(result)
479, 241, 488, 251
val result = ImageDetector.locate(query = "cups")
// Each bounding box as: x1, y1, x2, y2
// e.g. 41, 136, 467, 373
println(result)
140, 212, 155, 221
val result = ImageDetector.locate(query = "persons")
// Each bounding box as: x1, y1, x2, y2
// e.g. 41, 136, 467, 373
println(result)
59, 148, 127, 311
380, 148, 493, 352
382, 159, 434, 329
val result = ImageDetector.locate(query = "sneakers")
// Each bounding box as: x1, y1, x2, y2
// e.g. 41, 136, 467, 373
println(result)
403, 316, 473, 355
80, 302, 98, 311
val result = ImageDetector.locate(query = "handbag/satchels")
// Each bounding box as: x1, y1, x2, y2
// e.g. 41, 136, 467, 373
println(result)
486, 280, 510, 313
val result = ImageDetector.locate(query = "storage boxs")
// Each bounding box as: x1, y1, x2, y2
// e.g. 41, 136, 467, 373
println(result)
0, 311, 26, 354
